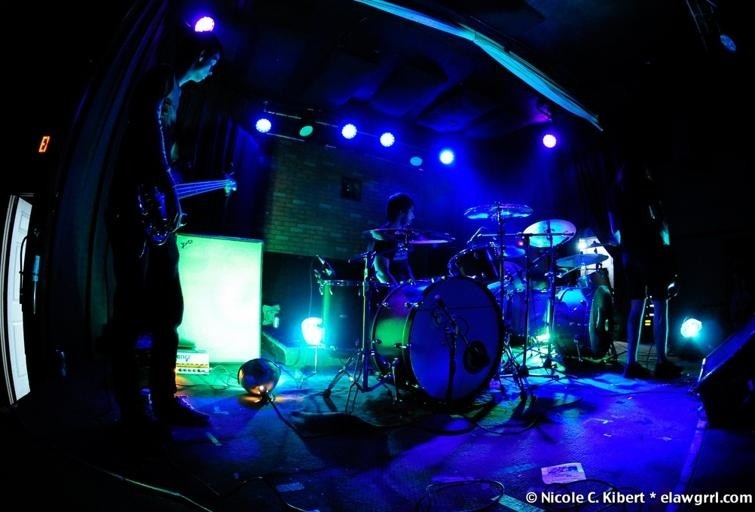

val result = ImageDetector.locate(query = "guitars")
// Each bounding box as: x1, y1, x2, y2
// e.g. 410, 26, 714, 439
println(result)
128, 171, 238, 246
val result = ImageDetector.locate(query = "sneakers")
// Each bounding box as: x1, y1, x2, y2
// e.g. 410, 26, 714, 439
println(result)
154, 396, 214, 428
624, 361, 651, 379
654, 361, 684, 380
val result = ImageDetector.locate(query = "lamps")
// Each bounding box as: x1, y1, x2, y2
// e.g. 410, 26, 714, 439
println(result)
256, 100, 395, 149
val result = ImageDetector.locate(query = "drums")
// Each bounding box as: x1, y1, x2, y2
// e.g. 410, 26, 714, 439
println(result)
575, 269, 615, 302
369, 275, 504, 409
505, 288, 548, 338
543, 285, 614, 358
447, 242, 512, 291
319, 278, 393, 358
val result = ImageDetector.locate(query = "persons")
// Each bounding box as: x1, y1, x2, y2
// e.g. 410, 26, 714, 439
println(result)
362, 192, 418, 317
608, 147, 687, 379
105, 31, 223, 429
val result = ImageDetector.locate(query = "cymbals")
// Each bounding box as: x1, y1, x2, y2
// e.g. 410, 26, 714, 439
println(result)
584, 242, 609, 249
464, 202, 532, 219
524, 219, 576, 248
361, 228, 417, 242
556, 253, 607, 270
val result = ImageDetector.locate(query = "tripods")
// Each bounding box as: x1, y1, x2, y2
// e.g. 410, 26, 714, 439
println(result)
492, 221, 576, 403
320, 244, 401, 415
